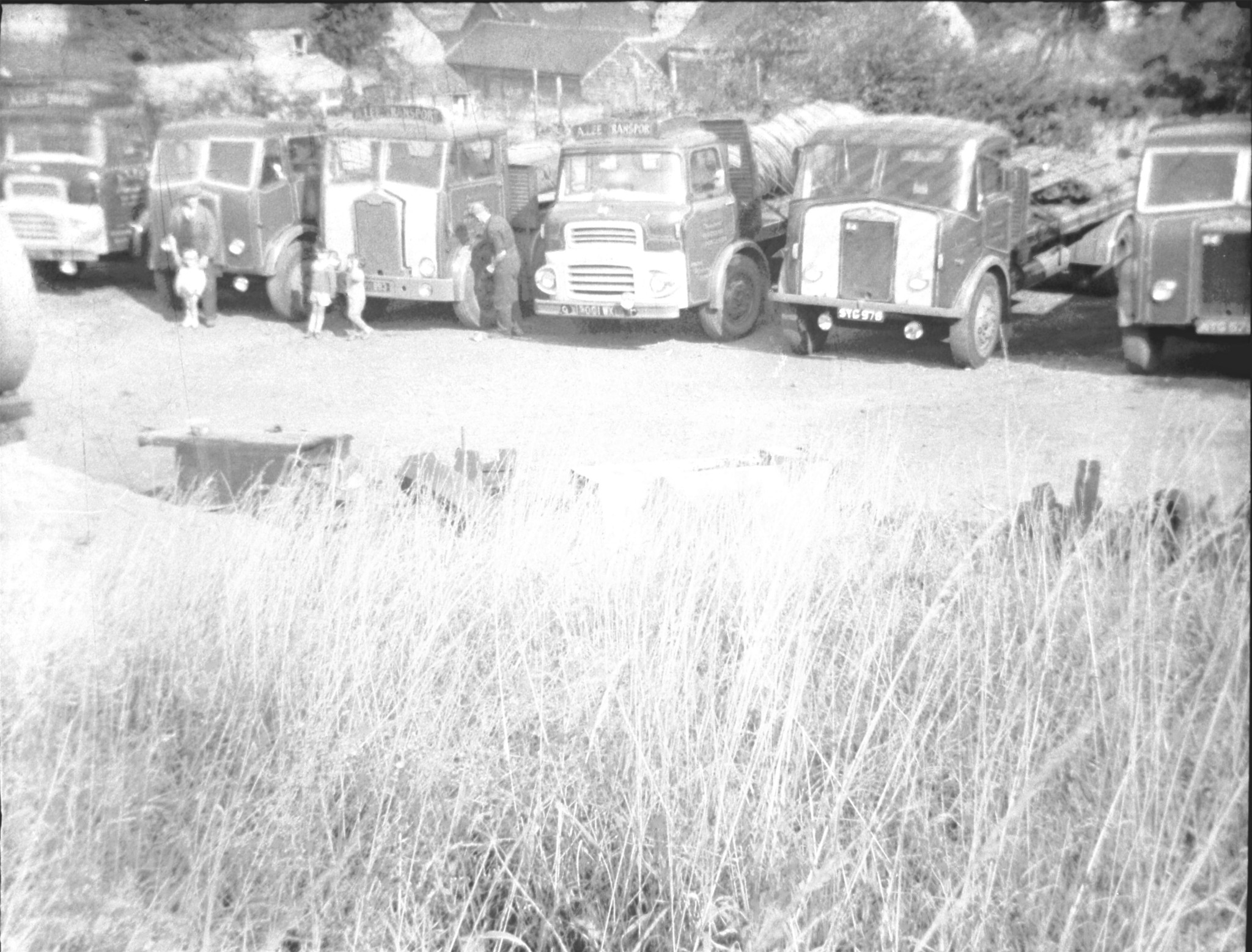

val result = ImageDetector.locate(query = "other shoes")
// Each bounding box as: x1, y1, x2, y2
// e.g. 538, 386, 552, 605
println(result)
347, 333, 358, 340
304, 330, 313, 337
489, 328, 510, 339
182, 315, 191, 327
513, 329, 524, 336
314, 331, 323, 339
204, 318, 214, 327
361, 327, 373, 339
191, 317, 200, 328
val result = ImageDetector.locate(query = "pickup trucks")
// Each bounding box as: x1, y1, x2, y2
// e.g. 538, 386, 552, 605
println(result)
766, 112, 1138, 371
528, 112, 792, 346
1115, 112, 1252, 375
141, 116, 328, 322
0, 78, 153, 286
306, 102, 539, 336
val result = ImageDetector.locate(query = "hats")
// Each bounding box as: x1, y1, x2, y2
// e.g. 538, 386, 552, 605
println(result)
176, 183, 199, 198
469, 202, 484, 212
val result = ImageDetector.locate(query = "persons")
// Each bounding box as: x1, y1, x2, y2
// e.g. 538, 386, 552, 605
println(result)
338, 254, 375, 340
163, 189, 219, 328
308, 250, 335, 339
468, 204, 524, 337
